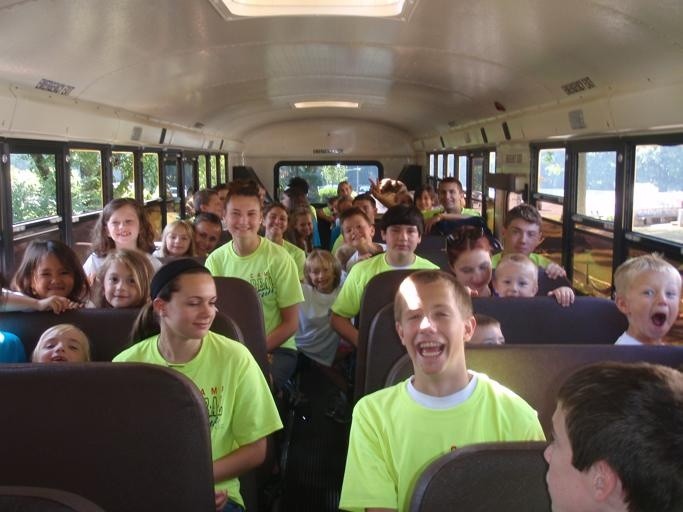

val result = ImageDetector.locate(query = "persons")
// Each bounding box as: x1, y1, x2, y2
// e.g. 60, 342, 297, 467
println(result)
294, 178, 577, 365
541, 361, 682, 511
335, 268, 546, 512
108, 254, 284, 511
611, 250, 682, 346
1, 174, 320, 364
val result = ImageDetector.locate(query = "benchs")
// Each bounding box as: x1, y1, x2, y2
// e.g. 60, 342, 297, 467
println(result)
70, 204, 510, 275
404, 443, 555, 512
385, 342, 683, 441
361, 299, 630, 400
208, 274, 273, 393
350, 266, 576, 403
3, 360, 217, 510
1, 306, 247, 368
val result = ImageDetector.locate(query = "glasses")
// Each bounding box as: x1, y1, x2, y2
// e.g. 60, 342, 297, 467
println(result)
447, 227, 483, 245
